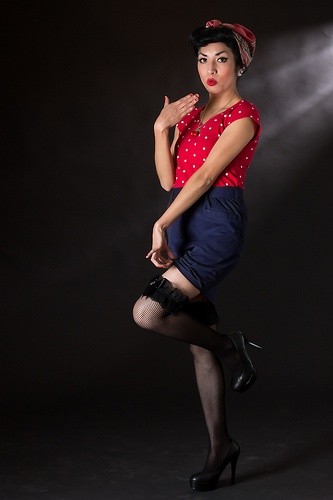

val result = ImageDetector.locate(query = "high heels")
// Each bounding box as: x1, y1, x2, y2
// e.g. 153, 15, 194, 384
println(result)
231, 331, 263, 391
190, 440, 241, 490
